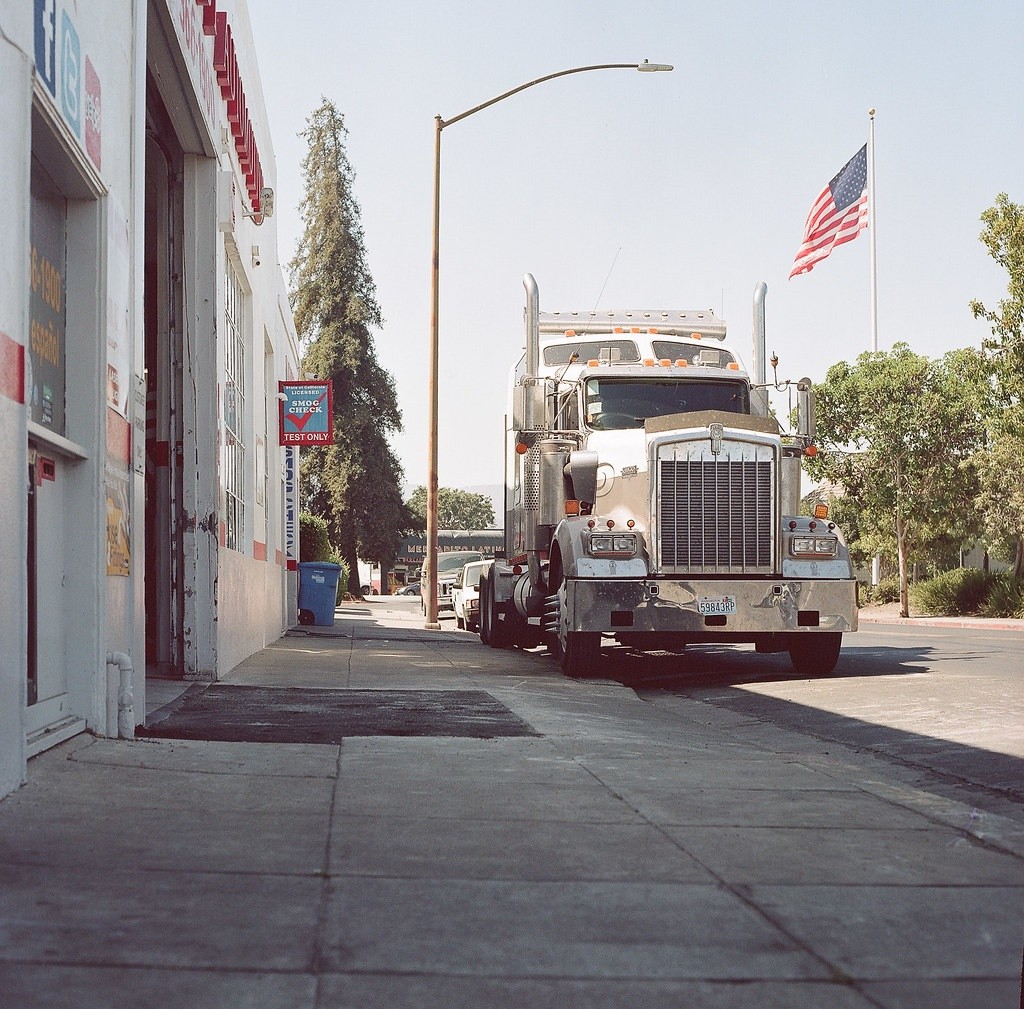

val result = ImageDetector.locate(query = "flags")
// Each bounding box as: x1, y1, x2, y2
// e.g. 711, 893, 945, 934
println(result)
788, 142, 870, 281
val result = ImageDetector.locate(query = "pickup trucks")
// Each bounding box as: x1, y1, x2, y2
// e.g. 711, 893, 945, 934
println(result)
451, 559, 505, 631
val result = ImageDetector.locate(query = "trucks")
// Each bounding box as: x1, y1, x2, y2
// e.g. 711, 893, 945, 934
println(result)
477, 271, 861, 682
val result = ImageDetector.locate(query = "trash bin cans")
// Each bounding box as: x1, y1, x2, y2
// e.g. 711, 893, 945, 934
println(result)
297, 561, 343, 626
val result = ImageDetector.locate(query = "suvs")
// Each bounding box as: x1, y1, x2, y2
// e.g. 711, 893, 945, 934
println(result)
421, 552, 486, 616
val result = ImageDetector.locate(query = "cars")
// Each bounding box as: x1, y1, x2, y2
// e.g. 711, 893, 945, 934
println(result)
403, 581, 420, 596
395, 585, 408, 594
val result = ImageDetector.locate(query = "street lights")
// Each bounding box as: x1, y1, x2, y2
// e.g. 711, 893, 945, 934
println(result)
419, 56, 680, 635
